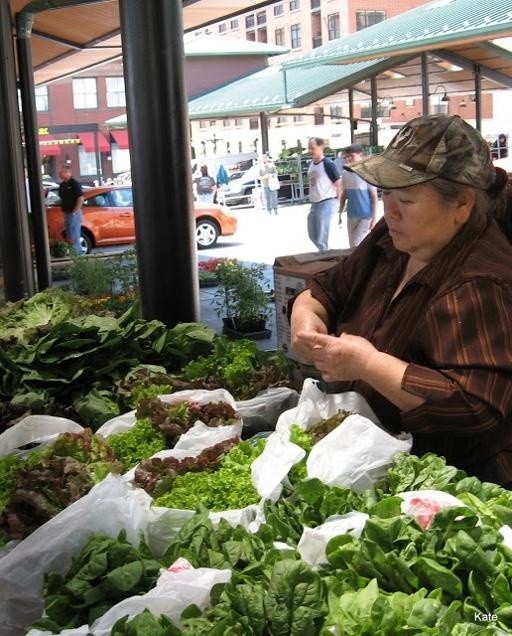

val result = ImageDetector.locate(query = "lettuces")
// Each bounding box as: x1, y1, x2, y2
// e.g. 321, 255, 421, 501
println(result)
0, 338, 312, 545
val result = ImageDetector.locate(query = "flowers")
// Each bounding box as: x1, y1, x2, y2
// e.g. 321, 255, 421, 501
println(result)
198, 256, 239, 275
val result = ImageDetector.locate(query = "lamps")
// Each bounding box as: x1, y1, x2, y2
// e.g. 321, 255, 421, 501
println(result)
430, 84, 451, 102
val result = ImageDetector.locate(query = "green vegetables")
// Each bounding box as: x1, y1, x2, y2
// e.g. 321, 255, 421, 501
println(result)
23, 453, 512, 636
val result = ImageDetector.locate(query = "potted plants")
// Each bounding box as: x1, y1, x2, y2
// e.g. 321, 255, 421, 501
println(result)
209, 259, 277, 333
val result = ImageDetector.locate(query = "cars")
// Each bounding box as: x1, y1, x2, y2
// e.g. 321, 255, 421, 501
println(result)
37, 184, 239, 257
41, 149, 316, 209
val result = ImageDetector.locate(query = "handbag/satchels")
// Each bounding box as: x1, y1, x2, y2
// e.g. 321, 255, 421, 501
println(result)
268, 174, 281, 191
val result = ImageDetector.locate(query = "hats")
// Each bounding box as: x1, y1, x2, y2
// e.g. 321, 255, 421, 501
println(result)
341, 114, 496, 192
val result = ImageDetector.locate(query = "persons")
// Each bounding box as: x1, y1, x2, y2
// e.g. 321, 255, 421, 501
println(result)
303, 136, 343, 250
57, 165, 85, 255
336, 143, 378, 251
191, 161, 216, 204
287, 110, 510, 487
489, 133, 508, 160
257, 154, 280, 216
